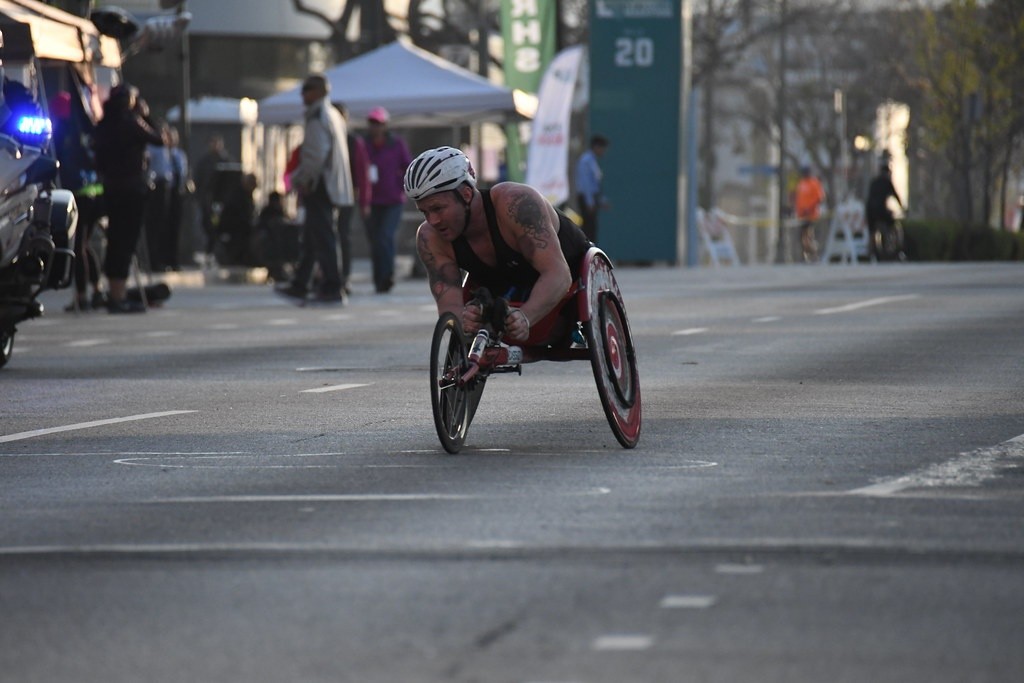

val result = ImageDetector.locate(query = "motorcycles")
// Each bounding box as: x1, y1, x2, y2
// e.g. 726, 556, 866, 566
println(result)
0, 132, 79, 370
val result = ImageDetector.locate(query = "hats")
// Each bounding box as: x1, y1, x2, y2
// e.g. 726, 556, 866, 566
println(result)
368, 106, 389, 124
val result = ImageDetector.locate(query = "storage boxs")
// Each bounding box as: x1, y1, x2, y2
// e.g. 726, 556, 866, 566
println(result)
36, 189, 79, 255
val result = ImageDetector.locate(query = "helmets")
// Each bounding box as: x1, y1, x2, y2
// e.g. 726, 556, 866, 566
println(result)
403, 145, 477, 202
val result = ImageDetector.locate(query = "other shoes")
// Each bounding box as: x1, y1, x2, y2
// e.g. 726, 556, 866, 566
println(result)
106, 294, 146, 313
91, 291, 106, 309
274, 284, 306, 306
64, 298, 90, 312
304, 289, 347, 308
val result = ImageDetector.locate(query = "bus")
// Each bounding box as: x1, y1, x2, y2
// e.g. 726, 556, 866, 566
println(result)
165, 95, 304, 218
0, 0, 121, 222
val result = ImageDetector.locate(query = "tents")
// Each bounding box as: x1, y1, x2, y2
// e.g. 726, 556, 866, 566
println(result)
259, 35, 538, 192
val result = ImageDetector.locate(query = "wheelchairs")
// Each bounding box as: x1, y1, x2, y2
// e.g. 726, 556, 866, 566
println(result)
428, 243, 642, 456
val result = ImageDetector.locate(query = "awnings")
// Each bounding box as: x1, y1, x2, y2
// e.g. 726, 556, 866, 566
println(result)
0, 0, 124, 68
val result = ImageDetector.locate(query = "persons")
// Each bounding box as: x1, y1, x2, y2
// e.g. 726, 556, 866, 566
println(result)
0, 26, 411, 314
794, 165, 826, 264
402, 145, 597, 343
867, 164, 907, 264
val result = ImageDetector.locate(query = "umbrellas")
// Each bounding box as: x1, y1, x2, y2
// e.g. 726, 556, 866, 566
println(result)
166, 94, 260, 127
